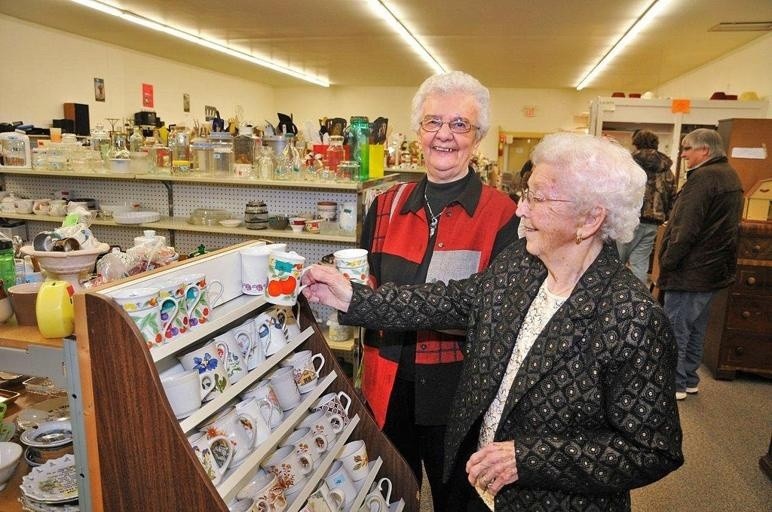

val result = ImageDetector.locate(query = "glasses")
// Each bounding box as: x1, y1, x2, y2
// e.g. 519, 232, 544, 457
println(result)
521, 188, 571, 211
420, 118, 480, 134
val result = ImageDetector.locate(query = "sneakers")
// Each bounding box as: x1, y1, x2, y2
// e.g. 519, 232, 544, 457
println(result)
686, 387, 698, 394
676, 392, 687, 400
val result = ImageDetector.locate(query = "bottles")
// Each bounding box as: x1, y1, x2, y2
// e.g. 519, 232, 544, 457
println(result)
338, 202, 358, 234
336, 161, 360, 183
189, 143, 213, 174
259, 144, 274, 179
210, 132, 234, 176
292, 156, 302, 181
304, 160, 319, 181
326, 135, 346, 170
320, 165, 333, 184
0, 240, 16, 294
172, 132, 190, 173
350, 117, 370, 181
282, 133, 298, 162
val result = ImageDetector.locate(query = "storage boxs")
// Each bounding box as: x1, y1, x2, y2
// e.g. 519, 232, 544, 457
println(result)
740, 178, 772, 224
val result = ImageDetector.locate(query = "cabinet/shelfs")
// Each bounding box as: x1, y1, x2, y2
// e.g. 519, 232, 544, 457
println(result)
701, 219, 772, 381
586, 95, 772, 191
0, 166, 402, 390
72, 238, 421, 512
717, 117, 772, 195
383, 166, 427, 184
0, 323, 76, 512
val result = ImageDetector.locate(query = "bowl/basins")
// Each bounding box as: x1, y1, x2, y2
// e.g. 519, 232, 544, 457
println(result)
109, 159, 130, 174
33, 199, 50, 215
19, 243, 111, 274
318, 209, 337, 220
0, 442, 23, 491
219, 220, 242, 228
318, 201, 338, 211
269, 214, 288, 229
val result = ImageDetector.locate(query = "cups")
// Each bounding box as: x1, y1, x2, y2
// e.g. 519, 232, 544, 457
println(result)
158, 369, 202, 419
229, 498, 270, 512
264, 252, 311, 306
309, 391, 351, 429
8, 282, 41, 325
15, 259, 26, 284
232, 319, 271, 371
214, 331, 251, 384
322, 461, 357, 505
368, 145, 384, 179
197, 406, 256, 468
254, 308, 290, 357
366, 478, 391, 512
111, 288, 179, 348
327, 311, 351, 341
14, 199, 33, 213
262, 366, 301, 411
233, 163, 253, 178
304, 218, 329, 233
289, 300, 301, 337
48, 199, 67, 216
288, 218, 306, 232
278, 427, 327, 461
186, 431, 233, 485
237, 473, 288, 511
239, 247, 272, 295
0, 198, 15, 214
63, 202, 88, 214
295, 411, 343, 449
175, 338, 231, 402
149, 281, 201, 336
231, 397, 272, 447
359, 496, 382, 512
238, 379, 284, 429
168, 273, 223, 326
333, 249, 369, 285
307, 480, 344, 512
279, 350, 325, 394
261, 445, 313, 495
334, 440, 370, 481
50, 127, 62, 142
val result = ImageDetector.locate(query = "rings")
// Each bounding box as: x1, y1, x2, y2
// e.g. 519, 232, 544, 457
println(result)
481, 477, 489, 484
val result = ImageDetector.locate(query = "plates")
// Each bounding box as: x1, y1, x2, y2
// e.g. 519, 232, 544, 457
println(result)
112, 210, 161, 224
20, 421, 74, 447
19, 454, 79, 504
24, 446, 43, 466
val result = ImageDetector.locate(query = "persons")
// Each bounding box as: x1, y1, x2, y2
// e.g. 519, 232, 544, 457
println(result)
361, 69, 521, 511
512, 159, 533, 189
301, 131, 685, 511
616, 129, 677, 282
659, 128, 744, 400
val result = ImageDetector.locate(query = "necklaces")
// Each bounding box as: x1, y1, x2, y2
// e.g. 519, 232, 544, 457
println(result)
424, 185, 449, 238
